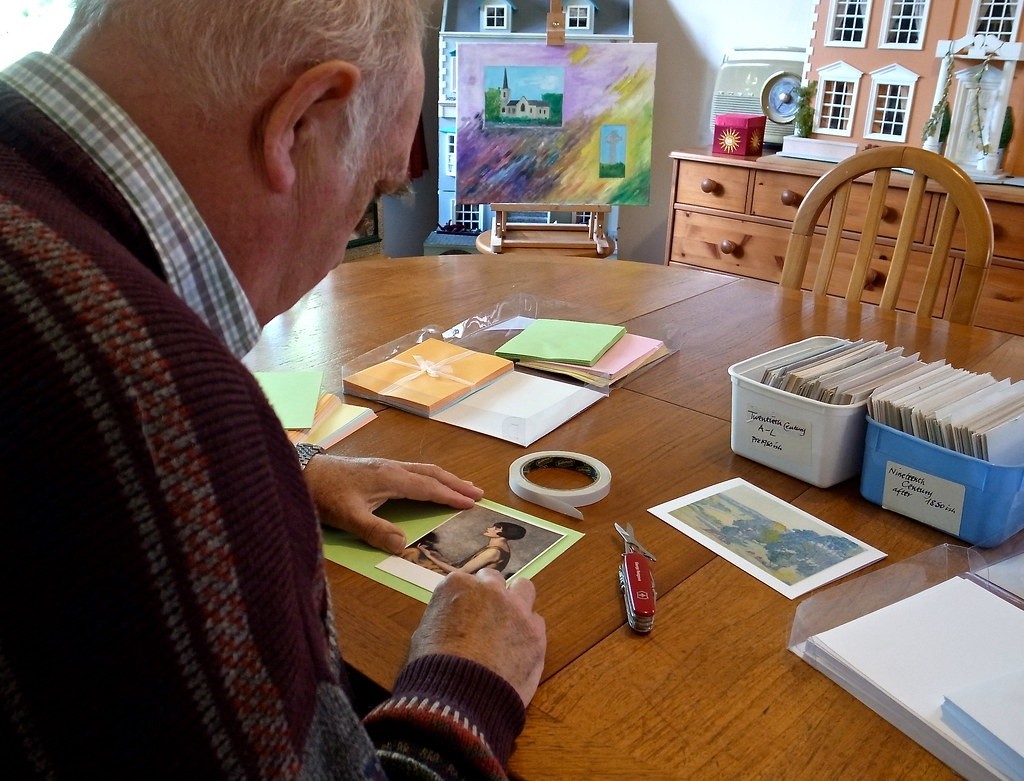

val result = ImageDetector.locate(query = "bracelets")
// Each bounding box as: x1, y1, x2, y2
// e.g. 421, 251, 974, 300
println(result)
296, 442, 326, 471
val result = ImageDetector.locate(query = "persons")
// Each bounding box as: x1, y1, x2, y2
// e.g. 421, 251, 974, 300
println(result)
402, 522, 527, 577
0, 0, 549, 780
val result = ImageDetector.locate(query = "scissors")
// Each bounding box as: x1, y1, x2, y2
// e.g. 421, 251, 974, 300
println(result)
614, 522, 657, 562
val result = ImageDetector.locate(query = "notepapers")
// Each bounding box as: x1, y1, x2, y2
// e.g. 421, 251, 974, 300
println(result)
253, 370, 379, 450
493, 319, 671, 387
343, 336, 515, 417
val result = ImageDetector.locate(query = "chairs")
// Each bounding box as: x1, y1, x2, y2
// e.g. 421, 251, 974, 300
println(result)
777, 147, 993, 326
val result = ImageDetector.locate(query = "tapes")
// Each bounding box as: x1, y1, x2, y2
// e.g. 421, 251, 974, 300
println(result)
508, 451, 612, 520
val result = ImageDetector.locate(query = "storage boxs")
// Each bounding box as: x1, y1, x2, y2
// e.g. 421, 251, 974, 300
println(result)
712, 112, 766, 157
728, 336, 928, 488
859, 413, 1024, 548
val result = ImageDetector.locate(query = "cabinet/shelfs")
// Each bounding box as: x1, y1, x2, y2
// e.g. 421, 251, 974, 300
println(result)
663, 147, 1024, 340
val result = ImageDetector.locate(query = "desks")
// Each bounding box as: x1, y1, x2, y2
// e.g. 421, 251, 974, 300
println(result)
236, 251, 1024, 781
476, 229, 615, 258
